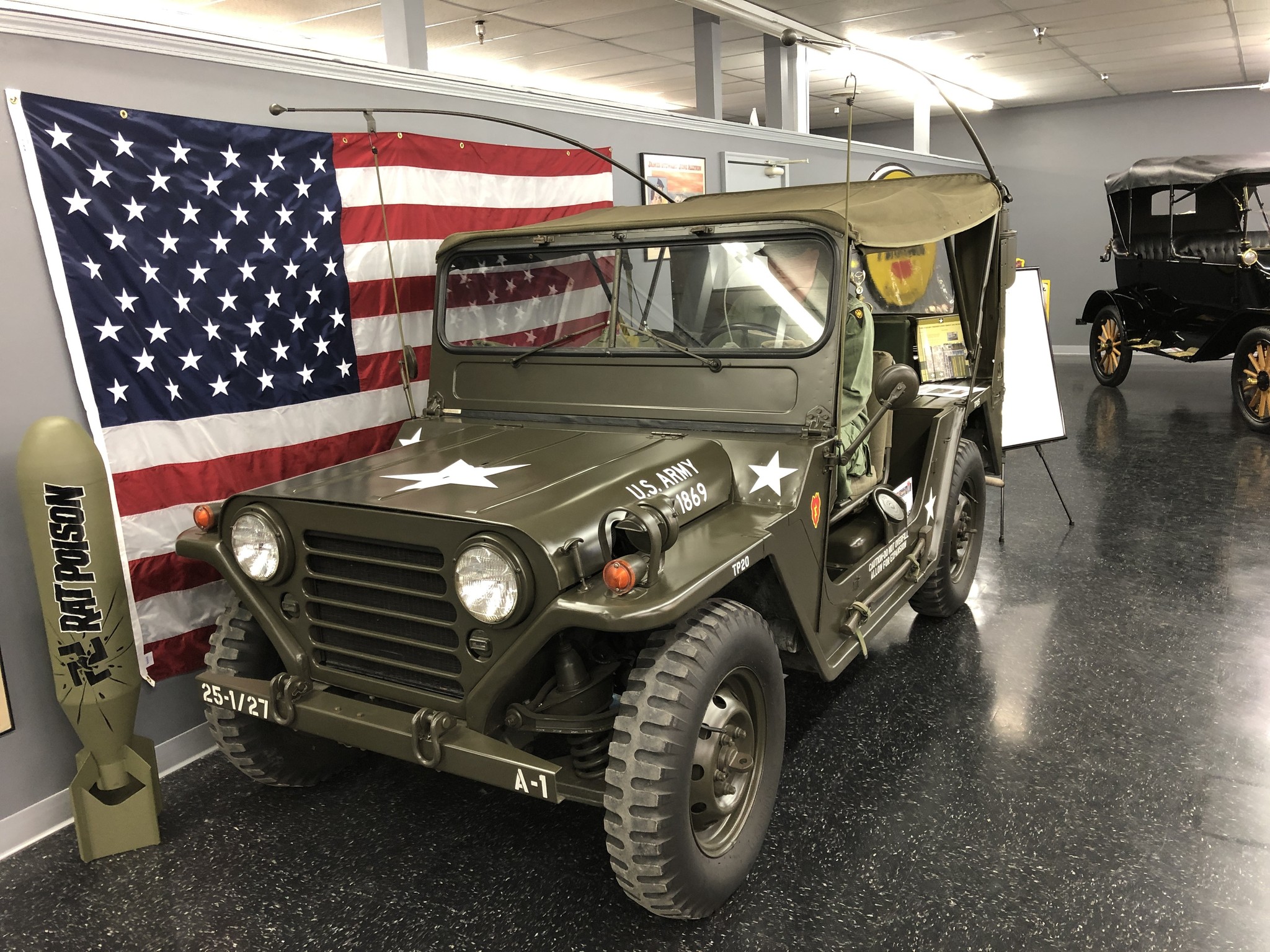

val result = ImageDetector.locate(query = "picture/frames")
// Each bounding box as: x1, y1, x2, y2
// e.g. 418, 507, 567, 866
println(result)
719, 151, 791, 192
639, 151, 707, 263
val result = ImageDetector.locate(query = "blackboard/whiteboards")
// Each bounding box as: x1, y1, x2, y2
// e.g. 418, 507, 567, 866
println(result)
1001, 266, 1069, 451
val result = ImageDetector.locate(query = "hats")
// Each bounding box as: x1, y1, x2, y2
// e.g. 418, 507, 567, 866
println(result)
754, 241, 820, 258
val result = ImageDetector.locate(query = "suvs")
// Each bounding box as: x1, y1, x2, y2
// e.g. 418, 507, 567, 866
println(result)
1072, 152, 1270, 437
171, 74, 1017, 922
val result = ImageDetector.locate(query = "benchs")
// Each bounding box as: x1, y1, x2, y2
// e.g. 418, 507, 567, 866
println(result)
873, 313, 920, 383
1111, 229, 1270, 266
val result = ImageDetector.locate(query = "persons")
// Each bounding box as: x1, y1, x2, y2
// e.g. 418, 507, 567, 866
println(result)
711, 240, 877, 503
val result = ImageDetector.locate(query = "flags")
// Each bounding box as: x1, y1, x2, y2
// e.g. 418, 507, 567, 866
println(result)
0, 87, 619, 688
